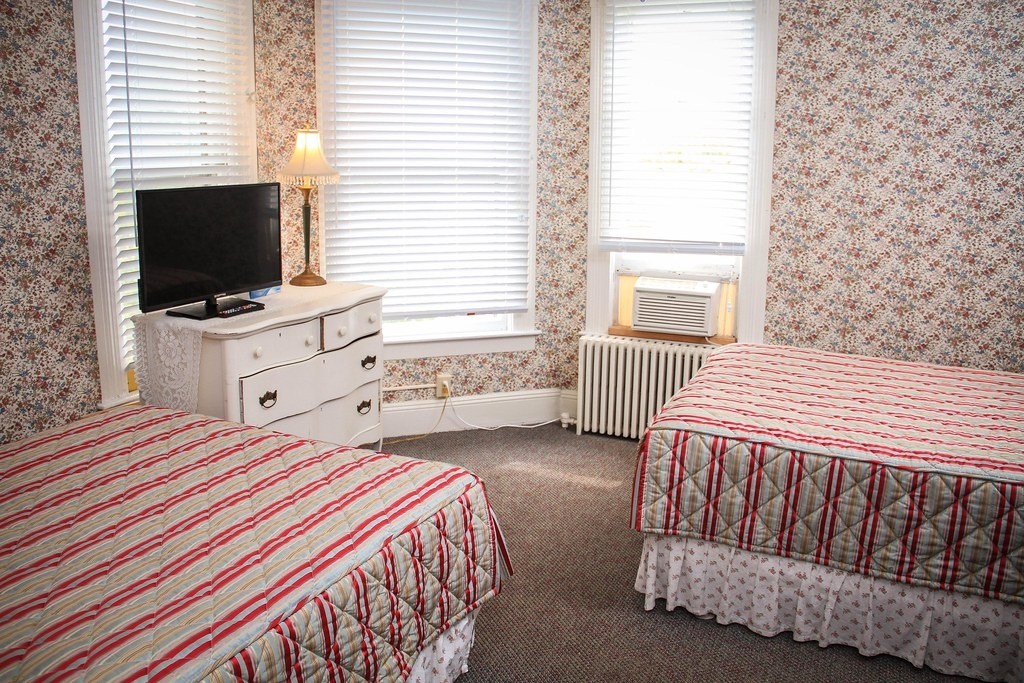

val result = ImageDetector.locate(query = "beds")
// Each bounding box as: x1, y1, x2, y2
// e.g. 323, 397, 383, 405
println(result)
618, 343, 1024, 683
0, 404, 517, 683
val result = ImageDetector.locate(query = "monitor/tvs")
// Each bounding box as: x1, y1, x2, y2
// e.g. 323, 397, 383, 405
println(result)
136, 181, 282, 320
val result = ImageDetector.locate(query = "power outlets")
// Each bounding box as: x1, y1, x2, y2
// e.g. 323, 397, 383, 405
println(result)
437, 375, 450, 397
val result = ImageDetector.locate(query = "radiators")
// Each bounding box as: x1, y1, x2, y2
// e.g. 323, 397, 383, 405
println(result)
575, 335, 717, 441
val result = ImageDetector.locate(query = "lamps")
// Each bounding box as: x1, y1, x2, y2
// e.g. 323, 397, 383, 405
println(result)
277, 128, 340, 287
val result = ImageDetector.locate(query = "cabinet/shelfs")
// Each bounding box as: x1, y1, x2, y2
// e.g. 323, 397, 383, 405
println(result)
129, 281, 388, 450
